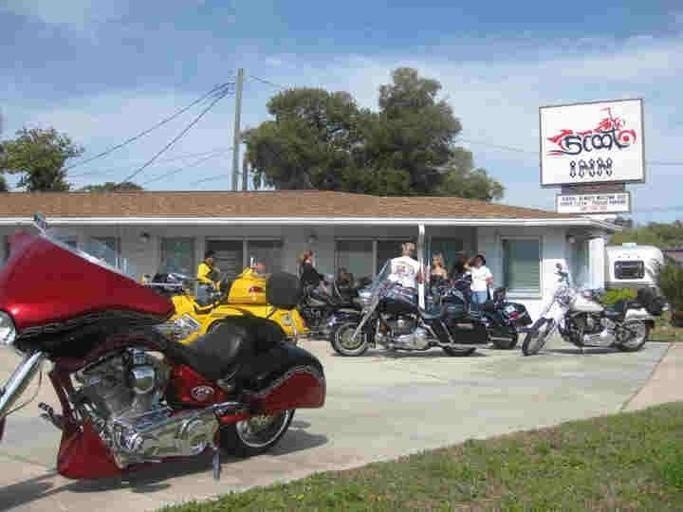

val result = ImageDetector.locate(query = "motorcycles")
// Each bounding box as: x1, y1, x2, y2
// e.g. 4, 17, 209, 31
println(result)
522, 262, 670, 356
0, 210, 326, 487
142, 248, 531, 358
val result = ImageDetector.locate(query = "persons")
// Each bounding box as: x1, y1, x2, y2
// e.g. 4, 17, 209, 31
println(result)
254, 255, 267, 275
462, 252, 493, 306
431, 250, 448, 280
196, 249, 230, 299
446, 250, 472, 312
382, 241, 422, 306
301, 249, 330, 300
